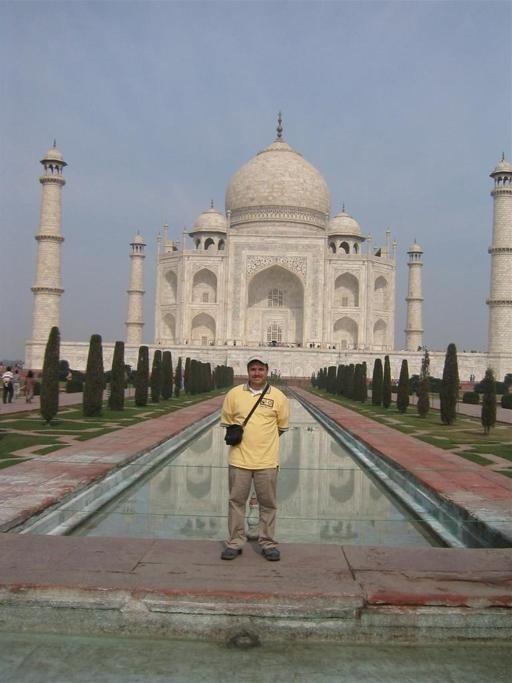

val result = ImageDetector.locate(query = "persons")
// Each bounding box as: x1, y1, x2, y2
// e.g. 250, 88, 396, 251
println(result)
1, 367, 35, 403
221, 355, 290, 561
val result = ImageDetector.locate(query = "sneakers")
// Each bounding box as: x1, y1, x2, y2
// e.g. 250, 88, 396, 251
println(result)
221, 548, 242, 560
263, 547, 280, 561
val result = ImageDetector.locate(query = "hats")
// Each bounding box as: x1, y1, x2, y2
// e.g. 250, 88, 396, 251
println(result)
248, 354, 267, 366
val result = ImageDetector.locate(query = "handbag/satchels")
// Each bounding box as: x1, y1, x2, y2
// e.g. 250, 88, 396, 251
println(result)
225, 424, 243, 446
